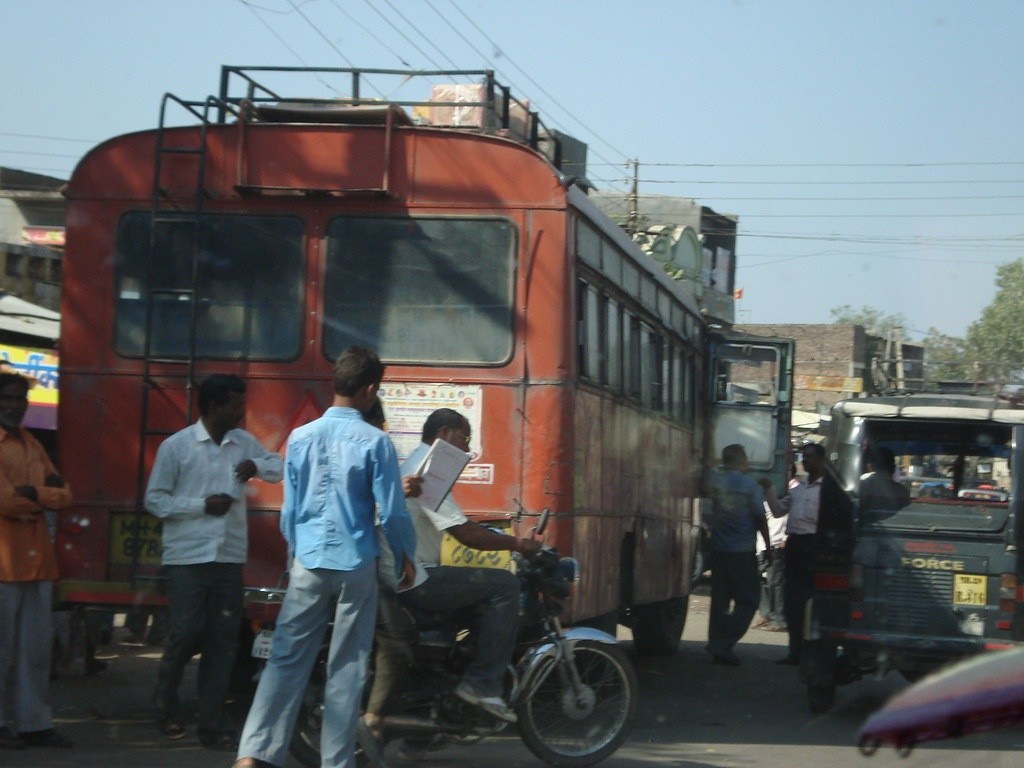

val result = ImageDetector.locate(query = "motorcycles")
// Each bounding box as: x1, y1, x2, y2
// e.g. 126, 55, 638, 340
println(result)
808, 400, 1024, 701
232, 500, 646, 768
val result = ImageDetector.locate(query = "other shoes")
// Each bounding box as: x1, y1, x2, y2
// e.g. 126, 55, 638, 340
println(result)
196, 730, 240, 751
355, 717, 388, 768
751, 617, 770, 629
773, 656, 799, 667
1, 729, 25, 749
23, 732, 75, 748
456, 679, 519, 723
765, 626, 788, 632
159, 715, 186, 739
706, 643, 740, 665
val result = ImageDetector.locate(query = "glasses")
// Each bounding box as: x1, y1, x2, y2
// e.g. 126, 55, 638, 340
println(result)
437, 426, 471, 444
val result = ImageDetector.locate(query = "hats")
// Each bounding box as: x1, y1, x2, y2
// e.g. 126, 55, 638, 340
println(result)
0, 364, 38, 390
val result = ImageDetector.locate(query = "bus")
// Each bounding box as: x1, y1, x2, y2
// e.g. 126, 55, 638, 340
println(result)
42, 56, 799, 690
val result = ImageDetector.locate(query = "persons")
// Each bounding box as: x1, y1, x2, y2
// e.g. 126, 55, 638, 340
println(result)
750, 501, 791, 630
363, 406, 549, 735
52, 610, 170, 679
702, 444, 772, 664
755, 443, 825, 662
233, 343, 421, 768
0, 372, 72, 746
142, 375, 285, 747
857, 447, 911, 499
